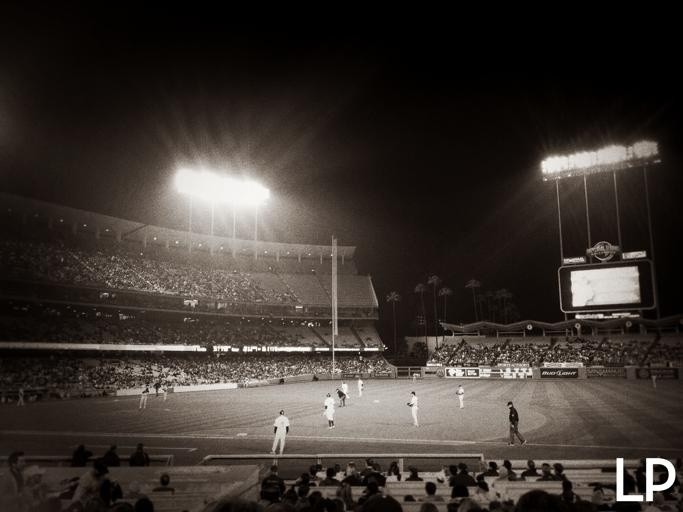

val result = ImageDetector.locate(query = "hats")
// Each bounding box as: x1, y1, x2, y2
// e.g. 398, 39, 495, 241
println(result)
23, 465, 46, 479
506, 401, 513, 406
410, 391, 415, 395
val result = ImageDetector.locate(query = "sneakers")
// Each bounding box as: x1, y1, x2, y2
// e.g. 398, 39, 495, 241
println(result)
328, 425, 335, 431
507, 441, 515, 446
520, 439, 527, 446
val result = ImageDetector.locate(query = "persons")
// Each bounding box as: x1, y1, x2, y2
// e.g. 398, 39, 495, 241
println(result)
0, 239, 683, 512
